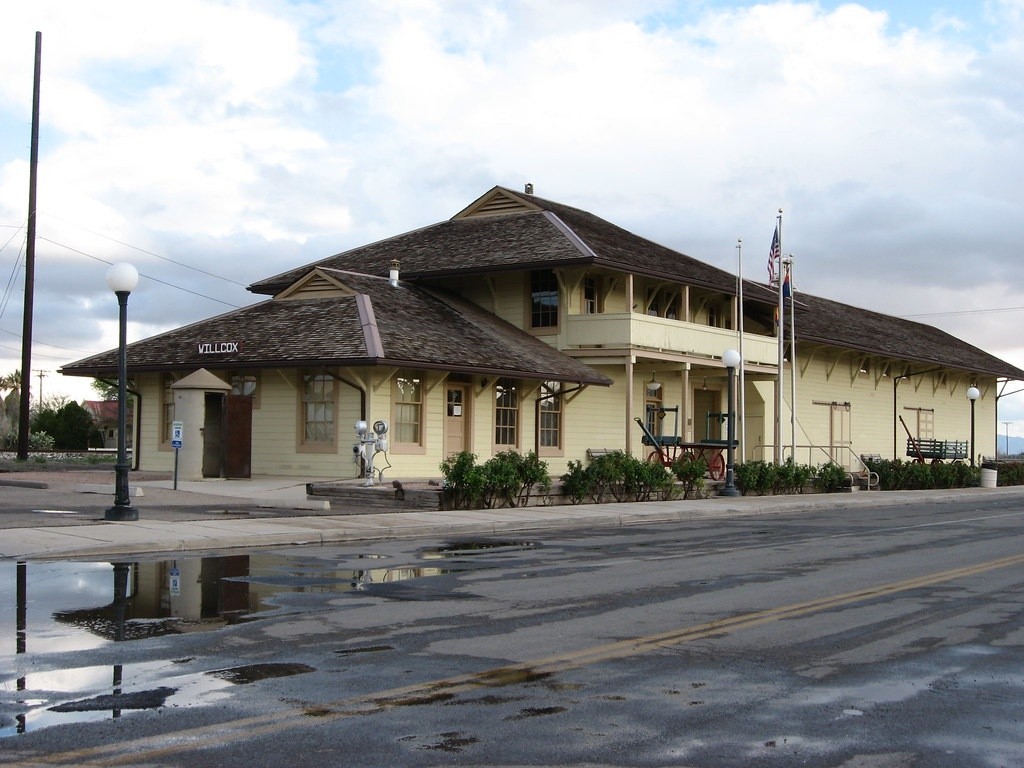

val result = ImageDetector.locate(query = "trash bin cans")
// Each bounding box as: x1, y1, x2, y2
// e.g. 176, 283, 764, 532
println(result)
979, 461, 1000, 489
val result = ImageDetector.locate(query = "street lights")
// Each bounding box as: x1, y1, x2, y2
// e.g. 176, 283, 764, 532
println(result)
104, 261, 141, 520
966, 387, 980, 468
718, 349, 743, 498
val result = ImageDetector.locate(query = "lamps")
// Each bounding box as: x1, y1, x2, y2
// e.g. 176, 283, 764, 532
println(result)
646, 370, 661, 390
702, 376, 707, 390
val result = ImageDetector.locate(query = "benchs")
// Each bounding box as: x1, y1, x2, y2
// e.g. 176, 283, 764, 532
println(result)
587, 448, 625, 464
861, 454, 889, 474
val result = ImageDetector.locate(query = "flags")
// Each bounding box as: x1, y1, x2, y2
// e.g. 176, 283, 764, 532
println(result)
775, 270, 790, 326
766, 225, 780, 289
